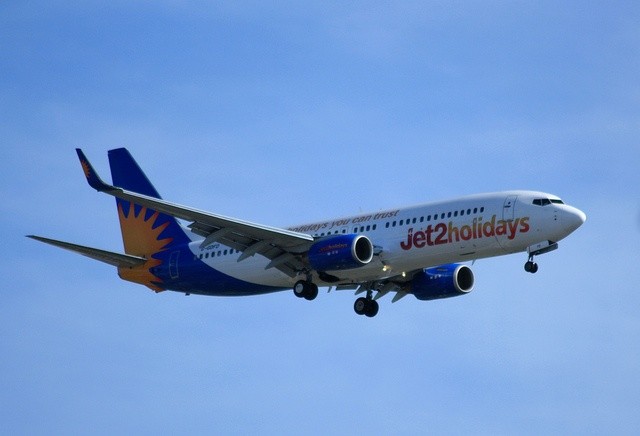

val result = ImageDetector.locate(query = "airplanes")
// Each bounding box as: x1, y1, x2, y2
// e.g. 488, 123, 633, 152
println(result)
24, 147, 587, 316
